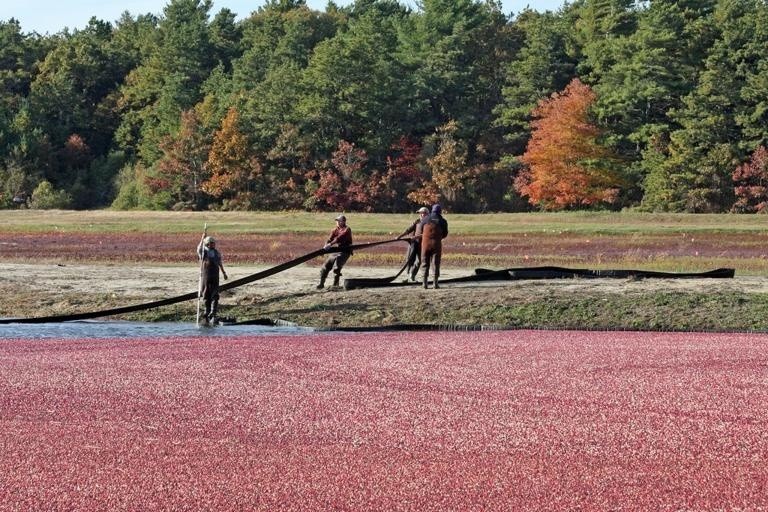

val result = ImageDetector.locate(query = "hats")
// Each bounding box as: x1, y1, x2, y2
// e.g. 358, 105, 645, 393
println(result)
335, 215, 346, 222
205, 236, 217, 245
416, 207, 429, 213
432, 204, 442, 212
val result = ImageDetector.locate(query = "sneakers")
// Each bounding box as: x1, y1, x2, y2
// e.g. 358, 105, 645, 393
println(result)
403, 276, 418, 283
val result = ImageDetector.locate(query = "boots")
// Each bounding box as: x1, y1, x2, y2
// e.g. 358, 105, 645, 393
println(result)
316, 276, 325, 289
432, 275, 440, 289
202, 302, 218, 318
333, 275, 339, 287
421, 275, 428, 289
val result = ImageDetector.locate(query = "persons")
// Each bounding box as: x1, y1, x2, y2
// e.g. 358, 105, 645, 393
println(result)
316, 215, 353, 288
196, 232, 228, 317
419, 203, 448, 288
394, 207, 430, 283
